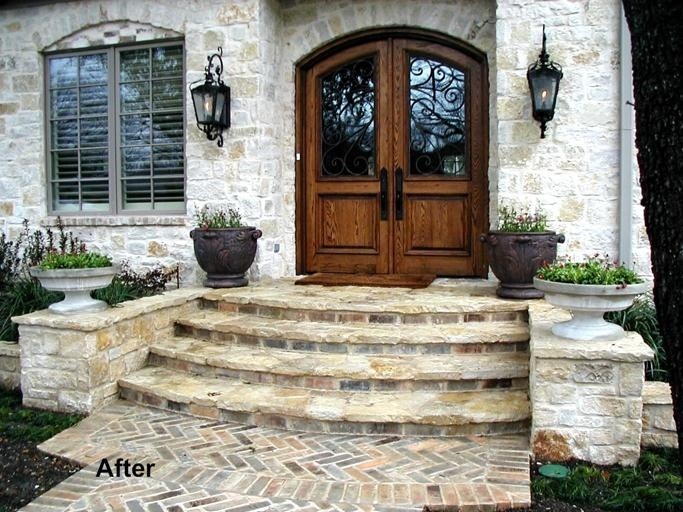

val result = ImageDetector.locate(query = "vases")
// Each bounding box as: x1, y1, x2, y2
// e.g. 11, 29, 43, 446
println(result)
188, 228, 264, 290
478, 229, 569, 300
531, 277, 649, 344
28, 265, 119, 314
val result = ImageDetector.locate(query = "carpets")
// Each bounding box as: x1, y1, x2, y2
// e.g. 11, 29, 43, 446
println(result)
294, 271, 437, 290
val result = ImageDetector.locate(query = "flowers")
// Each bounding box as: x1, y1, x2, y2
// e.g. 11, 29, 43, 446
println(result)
495, 200, 551, 235
534, 254, 644, 291
192, 202, 249, 228
35, 241, 115, 271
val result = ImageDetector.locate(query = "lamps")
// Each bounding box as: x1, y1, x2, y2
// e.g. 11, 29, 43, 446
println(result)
522, 21, 565, 141
186, 44, 232, 149
